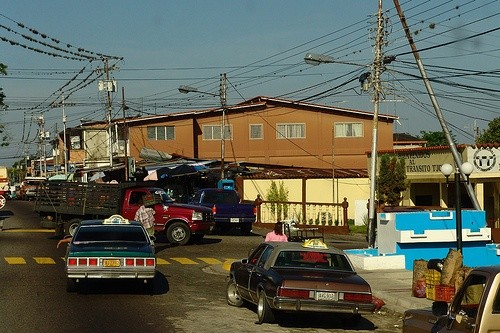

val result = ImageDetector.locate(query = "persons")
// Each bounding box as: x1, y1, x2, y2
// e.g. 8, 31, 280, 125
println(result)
265, 222, 288, 242
57, 237, 72, 248
134, 201, 156, 246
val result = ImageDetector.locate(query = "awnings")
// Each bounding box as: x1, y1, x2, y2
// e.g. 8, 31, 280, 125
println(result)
49, 174, 74, 181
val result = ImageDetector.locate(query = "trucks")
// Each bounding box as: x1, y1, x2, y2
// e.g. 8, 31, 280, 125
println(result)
34, 181, 219, 247
0, 166, 75, 209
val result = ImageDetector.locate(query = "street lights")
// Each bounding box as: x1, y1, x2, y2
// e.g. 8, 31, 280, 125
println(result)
179, 84, 229, 180
24, 141, 45, 177
302, 52, 384, 251
441, 164, 475, 269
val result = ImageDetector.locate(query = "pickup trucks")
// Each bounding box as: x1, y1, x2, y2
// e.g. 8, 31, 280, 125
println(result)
189, 188, 256, 235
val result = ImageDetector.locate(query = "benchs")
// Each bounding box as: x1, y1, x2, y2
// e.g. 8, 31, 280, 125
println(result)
274, 257, 330, 269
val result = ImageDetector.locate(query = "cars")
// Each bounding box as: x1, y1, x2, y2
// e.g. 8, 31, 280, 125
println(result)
431, 265, 500, 333
65, 215, 157, 285
226, 239, 377, 320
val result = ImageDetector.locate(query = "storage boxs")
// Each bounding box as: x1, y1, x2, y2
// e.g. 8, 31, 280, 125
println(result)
424, 268, 454, 302
